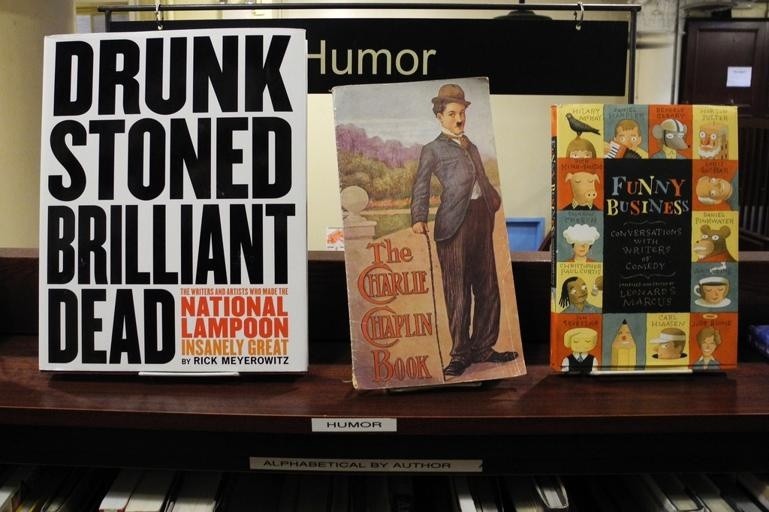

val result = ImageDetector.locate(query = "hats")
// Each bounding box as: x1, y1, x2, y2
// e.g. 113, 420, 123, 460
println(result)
431, 83, 472, 107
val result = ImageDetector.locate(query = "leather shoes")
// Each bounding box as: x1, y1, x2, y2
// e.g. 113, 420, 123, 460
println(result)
442, 360, 472, 377
472, 351, 518, 362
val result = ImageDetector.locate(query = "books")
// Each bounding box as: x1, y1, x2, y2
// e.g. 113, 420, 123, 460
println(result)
1, 470, 226, 512
643, 473, 769, 512
331, 74, 528, 390
450, 476, 570, 512
549, 96, 739, 374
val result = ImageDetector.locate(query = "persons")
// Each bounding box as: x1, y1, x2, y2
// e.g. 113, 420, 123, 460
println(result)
562, 327, 599, 366
412, 84, 518, 376
566, 136, 596, 158
560, 271, 601, 311
563, 221, 602, 263
650, 327, 685, 357
698, 122, 730, 160
610, 118, 647, 158
693, 325, 723, 363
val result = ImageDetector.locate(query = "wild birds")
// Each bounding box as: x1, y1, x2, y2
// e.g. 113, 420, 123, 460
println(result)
565, 111, 601, 139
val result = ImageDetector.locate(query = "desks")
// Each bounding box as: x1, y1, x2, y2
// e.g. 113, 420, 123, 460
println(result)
0, 331, 769, 434
